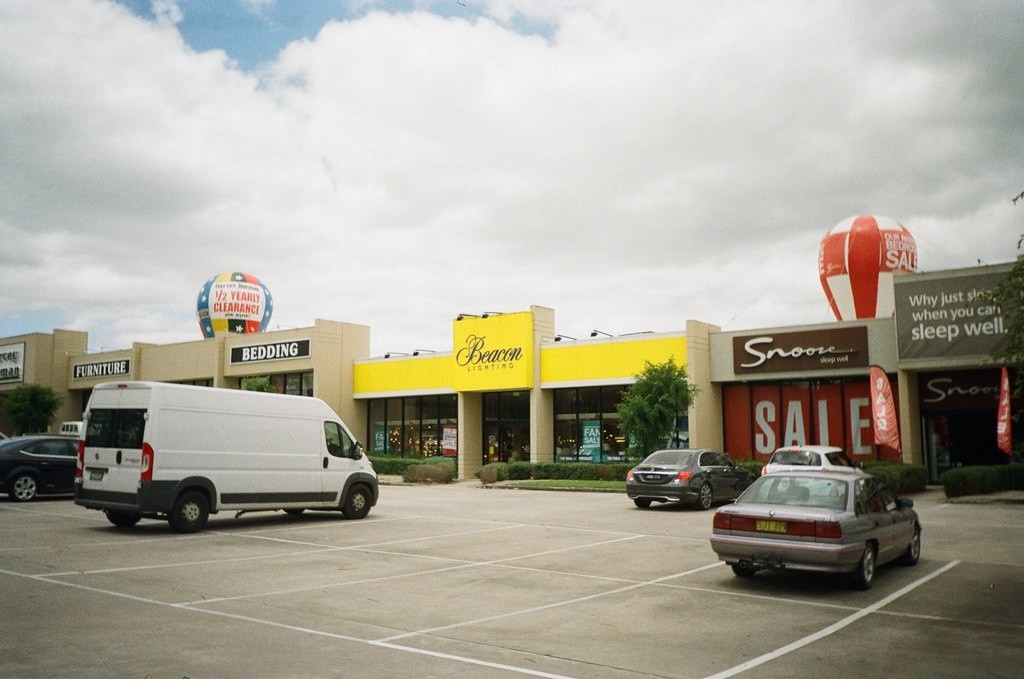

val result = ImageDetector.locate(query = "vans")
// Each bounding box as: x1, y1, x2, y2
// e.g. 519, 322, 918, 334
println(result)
74, 380, 379, 536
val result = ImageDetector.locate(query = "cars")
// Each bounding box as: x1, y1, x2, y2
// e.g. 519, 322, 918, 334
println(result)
763, 445, 864, 475
626, 449, 753, 510
0, 436, 83, 502
712, 471, 923, 593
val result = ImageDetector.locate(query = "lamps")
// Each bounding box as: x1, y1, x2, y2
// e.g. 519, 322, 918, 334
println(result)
413, 349, 436, 357
590, 329, 613, 338
482, 312, 502, 318
456, 313, 479, 321
554, 334, 577, 341
384, 351, 408, 358
